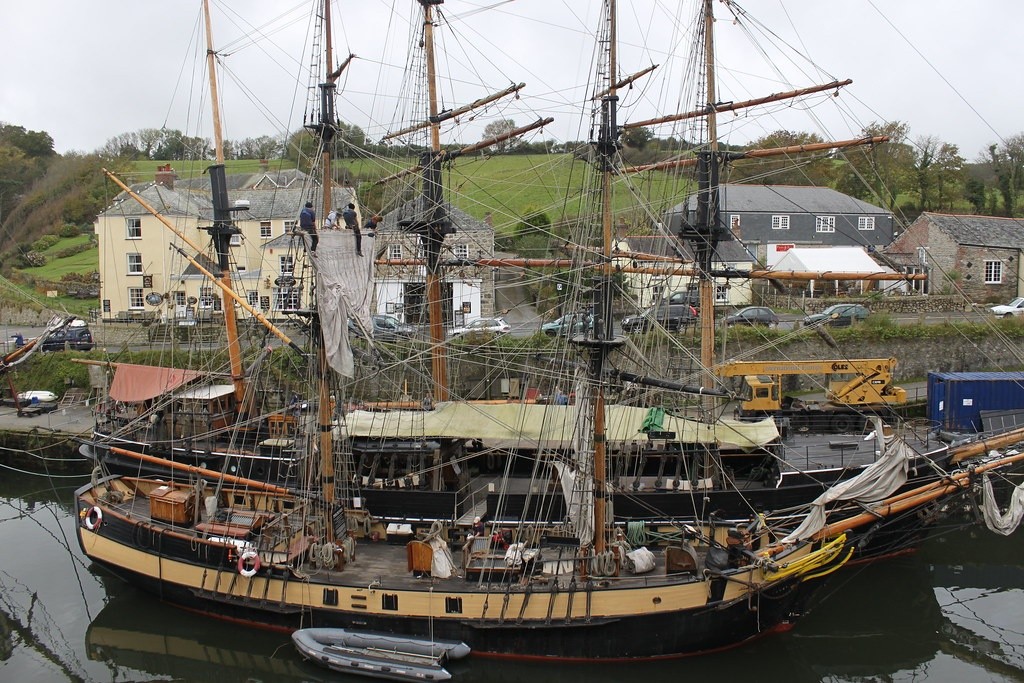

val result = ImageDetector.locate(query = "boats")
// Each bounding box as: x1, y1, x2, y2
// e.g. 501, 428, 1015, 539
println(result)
290, 627, 471, 682
17, 390, 58, 402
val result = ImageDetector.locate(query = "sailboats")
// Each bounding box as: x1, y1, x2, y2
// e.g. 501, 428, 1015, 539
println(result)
71, 1, 1024, 663
84, 596, 1024, 683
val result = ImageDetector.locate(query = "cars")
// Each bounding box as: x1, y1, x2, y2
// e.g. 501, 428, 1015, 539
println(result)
347, 315, 413, 337
662, 290, 699, 307
718, 306, 780, 329
621, 305, 697, 334
804, 304, 870, 330
446, 318, 512, 342
541, 311, 592, 337
990, 296, 1024, 319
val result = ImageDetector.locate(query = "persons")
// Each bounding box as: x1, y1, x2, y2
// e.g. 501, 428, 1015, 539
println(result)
364, 216, 383, 237
472, 516, 484, 536
300, 202, 319, 258
343, 203, 364, 257
324, 207, 343, 228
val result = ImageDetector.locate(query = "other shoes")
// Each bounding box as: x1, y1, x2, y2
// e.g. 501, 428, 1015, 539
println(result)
357, 251, 365, 257
312, 252, 318, 258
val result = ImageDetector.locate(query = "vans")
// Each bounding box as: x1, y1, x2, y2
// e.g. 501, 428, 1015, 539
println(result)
42, 326, 94, 352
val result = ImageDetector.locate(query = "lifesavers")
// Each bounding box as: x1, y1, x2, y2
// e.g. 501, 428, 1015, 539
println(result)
237, 551, 261, 578
85, 505, 103, 530
